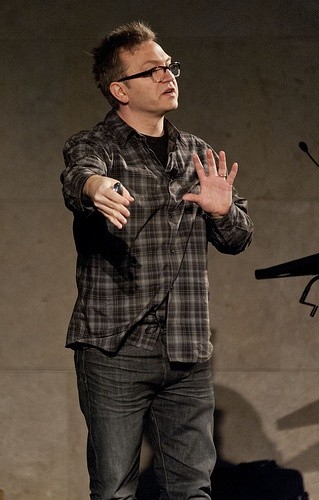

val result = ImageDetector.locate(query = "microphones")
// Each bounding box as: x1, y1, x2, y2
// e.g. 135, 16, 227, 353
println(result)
298, 141, 319, 168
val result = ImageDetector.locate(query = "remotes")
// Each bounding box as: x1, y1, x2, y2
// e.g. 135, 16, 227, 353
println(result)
114, 183, 123, 196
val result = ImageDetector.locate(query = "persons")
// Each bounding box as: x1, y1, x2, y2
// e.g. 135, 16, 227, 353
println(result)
56, 20, 254, 500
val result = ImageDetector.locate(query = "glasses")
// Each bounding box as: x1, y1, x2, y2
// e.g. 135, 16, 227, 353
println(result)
117, 61, 182, 83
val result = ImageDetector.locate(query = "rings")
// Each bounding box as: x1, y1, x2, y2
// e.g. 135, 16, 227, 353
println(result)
219, 175, 228, 177
